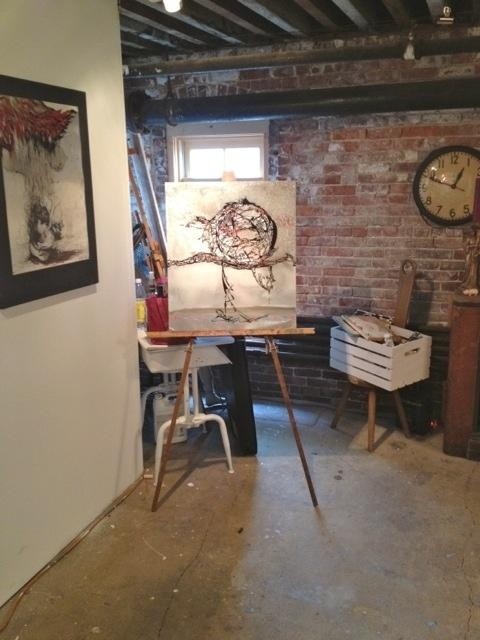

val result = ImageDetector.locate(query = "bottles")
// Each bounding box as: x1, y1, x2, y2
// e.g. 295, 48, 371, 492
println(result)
136, 278, 146, 328
149, 282, 165, 297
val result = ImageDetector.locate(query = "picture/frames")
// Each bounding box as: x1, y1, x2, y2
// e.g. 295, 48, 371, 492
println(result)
0, 76, 97, 309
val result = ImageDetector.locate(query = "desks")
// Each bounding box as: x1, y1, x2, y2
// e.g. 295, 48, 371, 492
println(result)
139, 332, 235, 485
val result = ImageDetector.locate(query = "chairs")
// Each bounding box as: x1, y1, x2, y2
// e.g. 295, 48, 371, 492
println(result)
329, 259, 412, 452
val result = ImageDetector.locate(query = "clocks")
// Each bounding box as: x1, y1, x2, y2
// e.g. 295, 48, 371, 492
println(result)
411, 145, 479, 224
